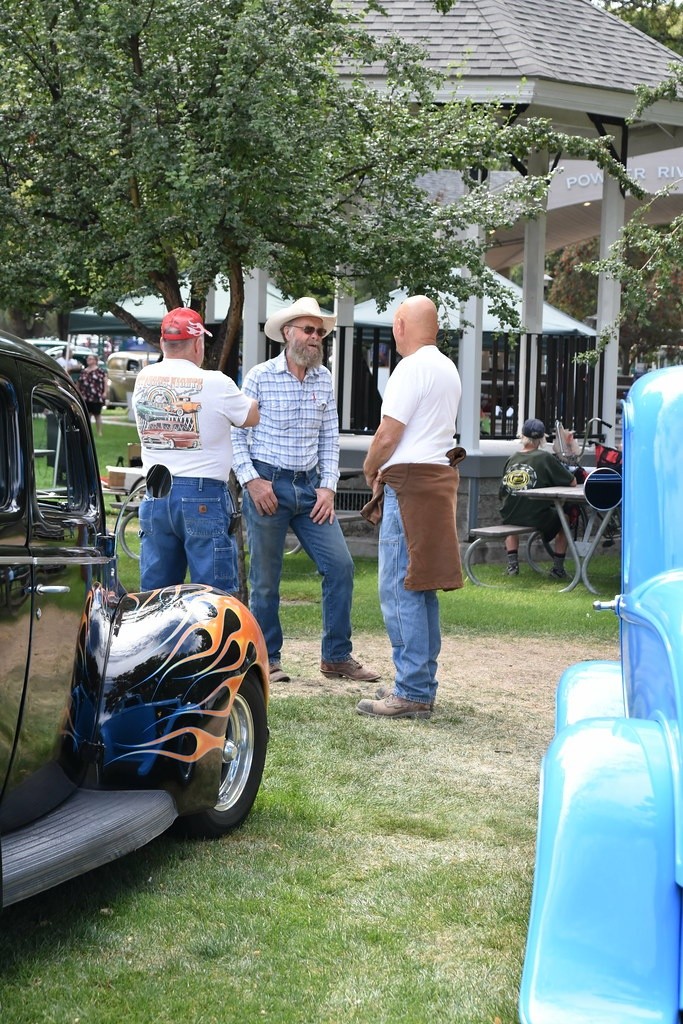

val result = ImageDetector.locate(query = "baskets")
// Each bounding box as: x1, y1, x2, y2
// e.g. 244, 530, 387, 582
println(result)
553, 452, 578, 466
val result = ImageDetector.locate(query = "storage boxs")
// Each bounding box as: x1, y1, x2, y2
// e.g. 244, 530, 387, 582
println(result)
107, 465, 144, 486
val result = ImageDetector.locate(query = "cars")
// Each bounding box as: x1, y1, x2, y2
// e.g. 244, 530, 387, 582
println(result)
21, 336, 162, 411
0, 328, 272, 915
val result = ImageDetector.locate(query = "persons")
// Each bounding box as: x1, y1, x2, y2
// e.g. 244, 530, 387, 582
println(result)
499, 418, 576, 582
55, 349, 82, 374
85, 338, 91, 347
231, 296, 381, 682
77, 356, 108, 436
132, 307, 261, 594
355, 295, 462, 717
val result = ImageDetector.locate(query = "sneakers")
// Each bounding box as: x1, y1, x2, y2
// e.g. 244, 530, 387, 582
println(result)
356, 693, 432, 720
549, 568, 571, 579
502, 564, 519, 575
377, 687, 434, 712
320, 658, 382, 681
268, 661, 291, 681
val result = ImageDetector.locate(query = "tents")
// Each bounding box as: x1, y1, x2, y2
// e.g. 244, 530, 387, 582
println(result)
53, 266, 329, 488
354, 267, 597, 337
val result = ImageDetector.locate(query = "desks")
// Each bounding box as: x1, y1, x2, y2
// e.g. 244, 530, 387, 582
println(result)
518, 484, 617, 595
285, 468, 365, 480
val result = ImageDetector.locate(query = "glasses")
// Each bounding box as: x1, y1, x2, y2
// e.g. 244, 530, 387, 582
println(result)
283, 325, 327, 336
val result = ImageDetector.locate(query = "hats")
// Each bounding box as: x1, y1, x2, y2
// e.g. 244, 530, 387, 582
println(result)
263, 296, 336, 343
522, 418, 545, 439
161, 307, 213, 340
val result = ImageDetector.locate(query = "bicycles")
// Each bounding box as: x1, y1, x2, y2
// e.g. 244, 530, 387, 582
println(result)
538, 417, 621, 560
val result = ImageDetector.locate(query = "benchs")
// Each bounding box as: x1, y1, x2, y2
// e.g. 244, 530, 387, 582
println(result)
109, 502, 367, 565
464, 525, 534, 586
